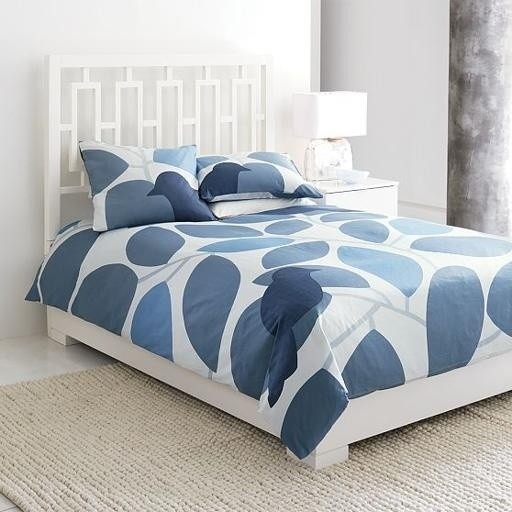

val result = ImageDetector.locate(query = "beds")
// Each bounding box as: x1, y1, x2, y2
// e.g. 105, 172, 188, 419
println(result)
24, 216, 512, 466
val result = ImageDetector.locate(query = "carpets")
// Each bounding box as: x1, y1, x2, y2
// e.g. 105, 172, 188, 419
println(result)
0, 364, 512, 512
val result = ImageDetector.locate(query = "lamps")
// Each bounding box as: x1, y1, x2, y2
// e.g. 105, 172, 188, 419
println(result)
294, 93, 377, 181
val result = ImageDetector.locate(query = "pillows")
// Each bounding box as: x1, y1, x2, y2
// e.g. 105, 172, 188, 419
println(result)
80, 136, 312, 234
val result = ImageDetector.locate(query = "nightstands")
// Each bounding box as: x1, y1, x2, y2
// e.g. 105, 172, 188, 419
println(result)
315, 183, 401, 215
